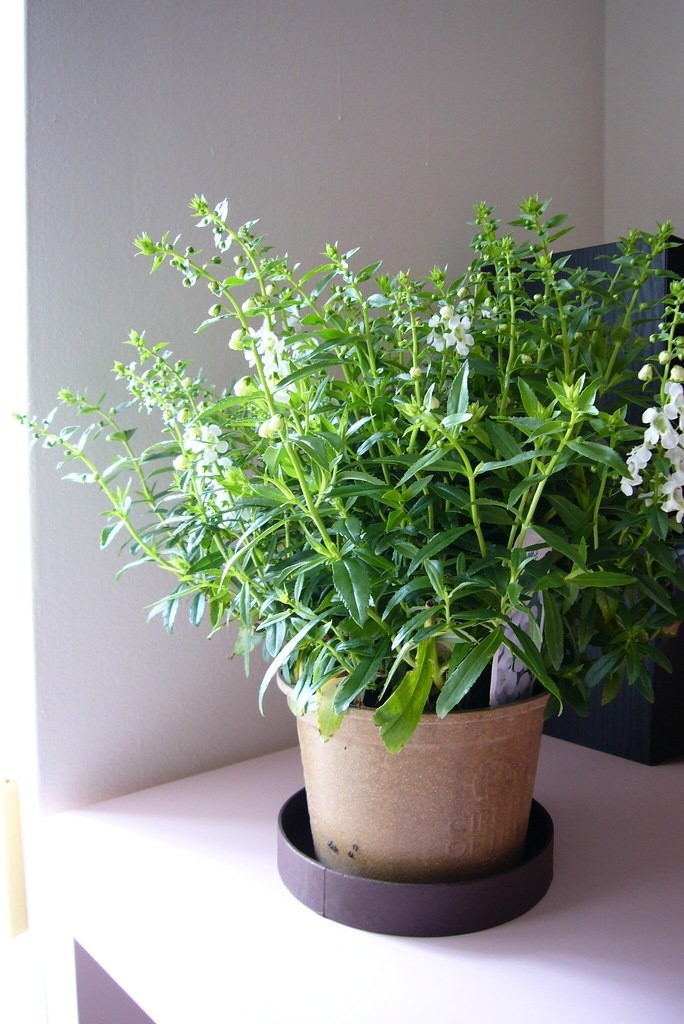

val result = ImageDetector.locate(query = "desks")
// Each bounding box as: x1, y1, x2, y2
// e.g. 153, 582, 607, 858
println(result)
32, 723, 684, 1024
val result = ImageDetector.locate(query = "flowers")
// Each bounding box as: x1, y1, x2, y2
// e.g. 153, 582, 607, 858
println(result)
9, 186, 684, 745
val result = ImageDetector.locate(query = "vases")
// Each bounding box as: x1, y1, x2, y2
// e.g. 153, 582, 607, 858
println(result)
275, 660, 557, 938
